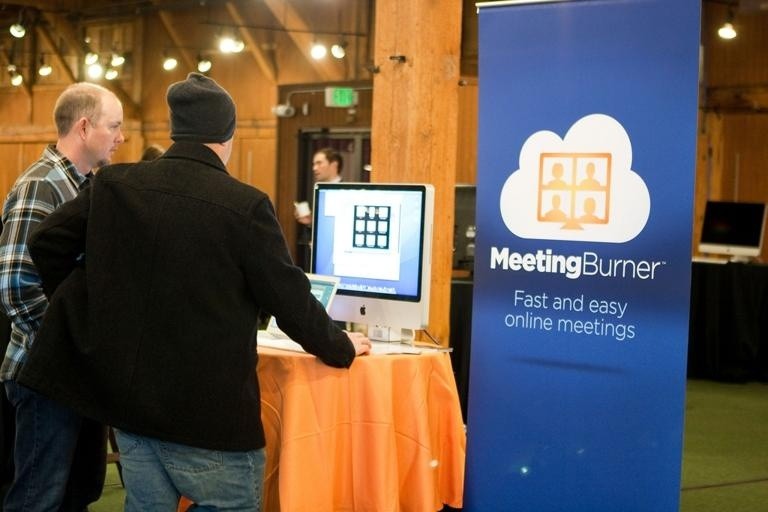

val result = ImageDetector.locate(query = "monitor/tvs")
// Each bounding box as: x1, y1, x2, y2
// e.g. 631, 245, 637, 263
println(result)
310, 182, 434, 355
697, 200, 765, 262
267, 272, 341, 337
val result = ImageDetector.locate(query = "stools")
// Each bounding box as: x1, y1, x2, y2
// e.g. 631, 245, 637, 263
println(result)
102, 425, 125, 488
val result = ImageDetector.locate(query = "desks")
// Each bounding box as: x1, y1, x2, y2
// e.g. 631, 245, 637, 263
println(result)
176, 341, 466, 512
692, 261, 768, 383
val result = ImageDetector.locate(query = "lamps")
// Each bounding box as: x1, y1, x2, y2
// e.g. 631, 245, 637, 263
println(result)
717, 2, 740, 40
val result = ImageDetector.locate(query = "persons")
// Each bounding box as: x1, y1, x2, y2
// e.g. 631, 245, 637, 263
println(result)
293, 146, 347, 332
1, 81, 124, 510
16, 70, 372, 511
141, 146, 165, 161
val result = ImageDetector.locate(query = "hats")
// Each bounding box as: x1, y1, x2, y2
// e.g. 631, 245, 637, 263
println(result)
166, 72, 236, 144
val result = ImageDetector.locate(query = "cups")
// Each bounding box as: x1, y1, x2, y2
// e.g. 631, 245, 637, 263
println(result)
294, 201, 311, 217
